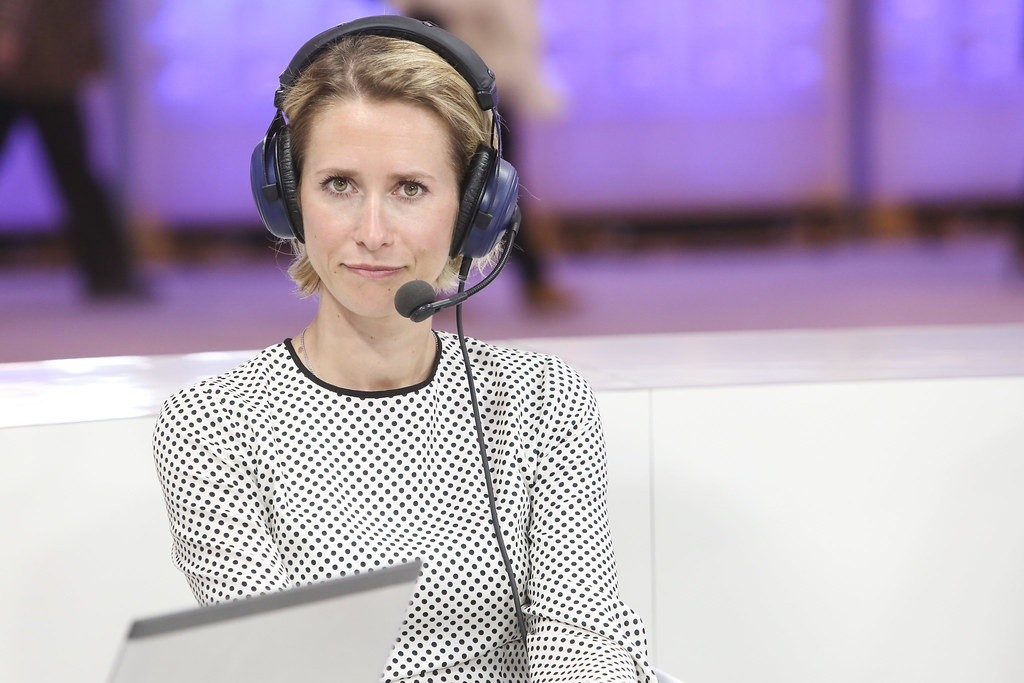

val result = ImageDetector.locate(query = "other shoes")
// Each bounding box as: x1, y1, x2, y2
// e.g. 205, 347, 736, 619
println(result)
524, 285, 579, 316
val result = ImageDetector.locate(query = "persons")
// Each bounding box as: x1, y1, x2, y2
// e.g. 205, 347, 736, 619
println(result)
149, 14, 684, 683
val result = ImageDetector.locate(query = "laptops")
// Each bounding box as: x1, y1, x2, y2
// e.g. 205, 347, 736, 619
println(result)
108, 560, 427, 683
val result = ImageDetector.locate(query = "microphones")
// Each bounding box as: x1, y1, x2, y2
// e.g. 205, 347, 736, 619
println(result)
394, 207, 521, 323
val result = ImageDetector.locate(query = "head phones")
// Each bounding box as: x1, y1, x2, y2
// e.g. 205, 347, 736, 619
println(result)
251, 15, 519, 260
